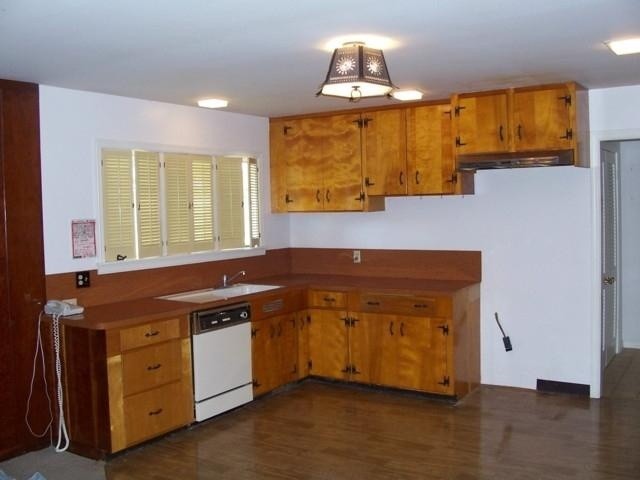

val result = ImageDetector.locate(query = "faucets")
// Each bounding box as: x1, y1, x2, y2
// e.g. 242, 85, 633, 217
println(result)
222, 270, 246, 286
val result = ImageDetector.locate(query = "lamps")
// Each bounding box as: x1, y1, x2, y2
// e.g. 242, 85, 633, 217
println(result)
315, 40, 399, 103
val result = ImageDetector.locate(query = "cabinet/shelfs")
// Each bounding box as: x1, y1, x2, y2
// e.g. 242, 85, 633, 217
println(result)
44, 314, 195, 461
347, 286, 480, 400
269, 109, 385, 213
361, 97, 475, 196
453, 81, 590, 169
300, 290, 350, 383
247, 286, 308, 398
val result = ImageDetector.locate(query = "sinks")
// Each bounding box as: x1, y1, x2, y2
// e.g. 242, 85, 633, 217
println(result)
212, 284, 280, 296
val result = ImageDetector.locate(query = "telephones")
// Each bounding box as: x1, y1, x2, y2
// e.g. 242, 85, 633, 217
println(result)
44, 299, 84, 315
